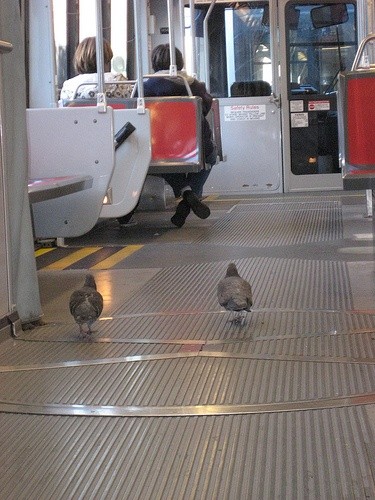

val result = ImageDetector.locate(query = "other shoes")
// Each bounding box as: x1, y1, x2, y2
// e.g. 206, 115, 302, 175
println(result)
120, 216, 137, 227
183, 189, 211, 220
170, 200, 190, 227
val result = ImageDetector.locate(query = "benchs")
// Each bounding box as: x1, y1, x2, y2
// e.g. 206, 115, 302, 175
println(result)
337, 33, 375, 218
27, 73, 227, 249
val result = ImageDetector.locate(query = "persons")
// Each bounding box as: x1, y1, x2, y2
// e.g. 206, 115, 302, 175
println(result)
133, 44, 217, 228
60, 37, 133, 225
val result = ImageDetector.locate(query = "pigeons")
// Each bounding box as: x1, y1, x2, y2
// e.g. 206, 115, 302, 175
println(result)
216, 261, 254, 325
68, 271, 104, 339
